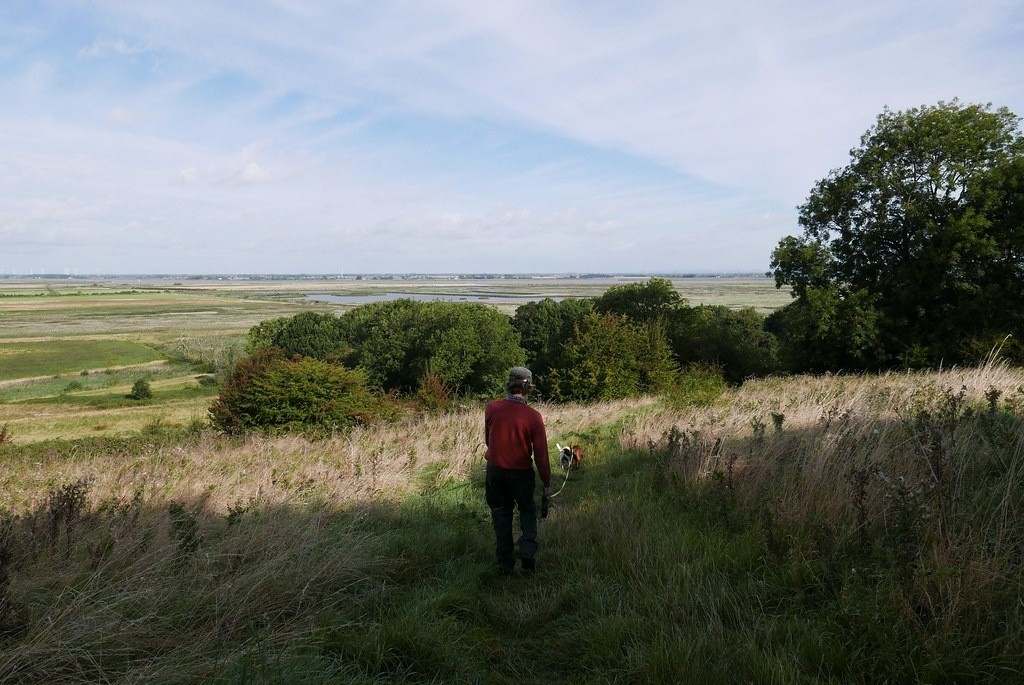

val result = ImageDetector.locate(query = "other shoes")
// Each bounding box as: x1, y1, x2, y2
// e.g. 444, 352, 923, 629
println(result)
522, 559, 535, 577
499, 561, 514, 574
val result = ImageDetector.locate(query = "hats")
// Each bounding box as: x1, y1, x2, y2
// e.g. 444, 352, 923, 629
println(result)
507, 367, 535, 387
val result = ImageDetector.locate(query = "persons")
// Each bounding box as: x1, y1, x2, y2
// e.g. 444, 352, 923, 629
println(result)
484, 367, 551, 578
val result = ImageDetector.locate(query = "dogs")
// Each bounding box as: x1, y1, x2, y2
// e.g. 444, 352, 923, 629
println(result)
556, 442, 583, 471
